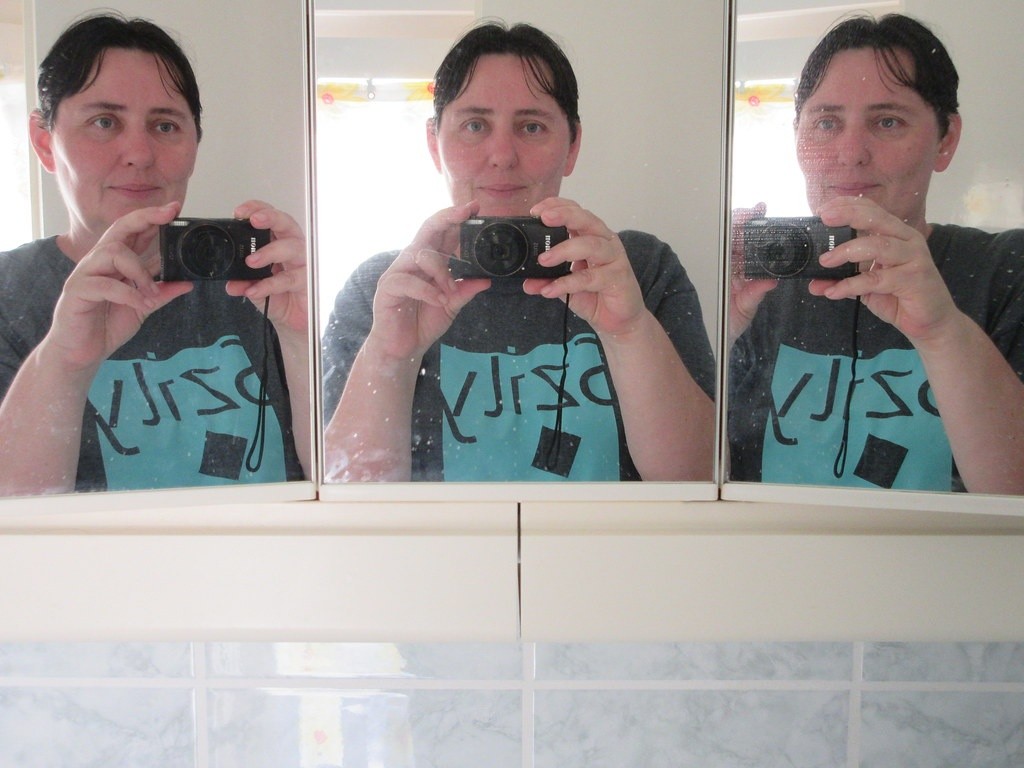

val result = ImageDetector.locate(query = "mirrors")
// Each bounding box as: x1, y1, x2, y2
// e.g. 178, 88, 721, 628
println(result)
0, 0, 1024, 500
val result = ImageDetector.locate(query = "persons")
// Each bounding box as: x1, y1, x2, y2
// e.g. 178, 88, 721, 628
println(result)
726, 9, 1023, 495
0, 7, 312, 501
323, 15, 721, 486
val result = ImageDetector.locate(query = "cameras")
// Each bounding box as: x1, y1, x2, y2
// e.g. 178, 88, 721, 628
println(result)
460, 214, 568, 280
158, 218, 272, 282
745, 214, 860, 282
